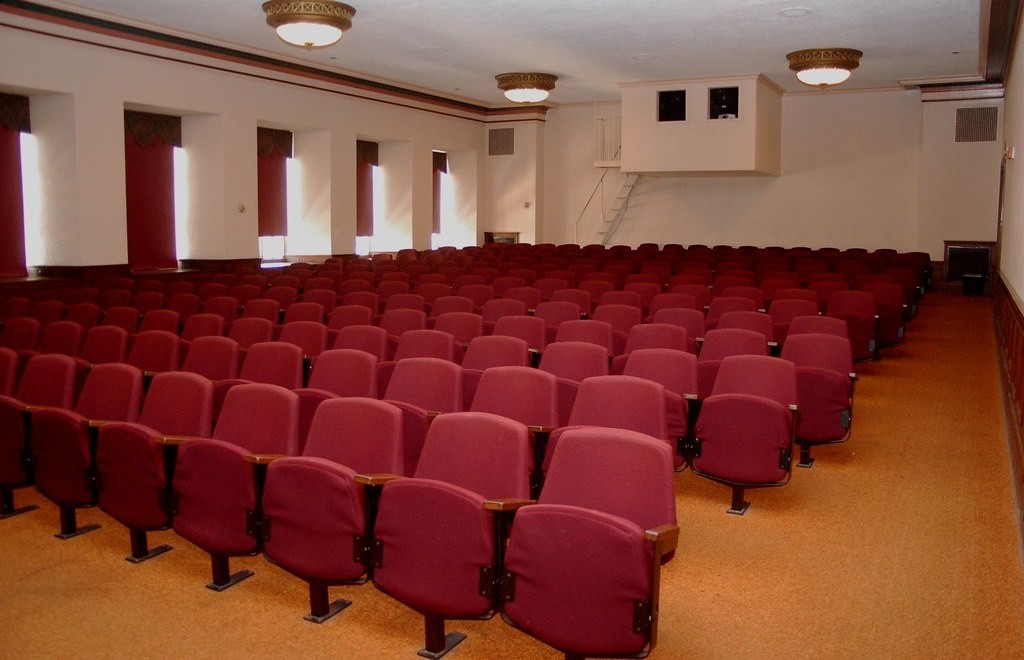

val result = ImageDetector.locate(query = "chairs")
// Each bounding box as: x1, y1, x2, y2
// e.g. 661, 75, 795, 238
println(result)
0, 241, 936, 660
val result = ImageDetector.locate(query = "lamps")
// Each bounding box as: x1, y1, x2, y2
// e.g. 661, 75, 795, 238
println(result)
263, 0, 356, 49
495, 72, 558, 103
786, 48, 864, 88
